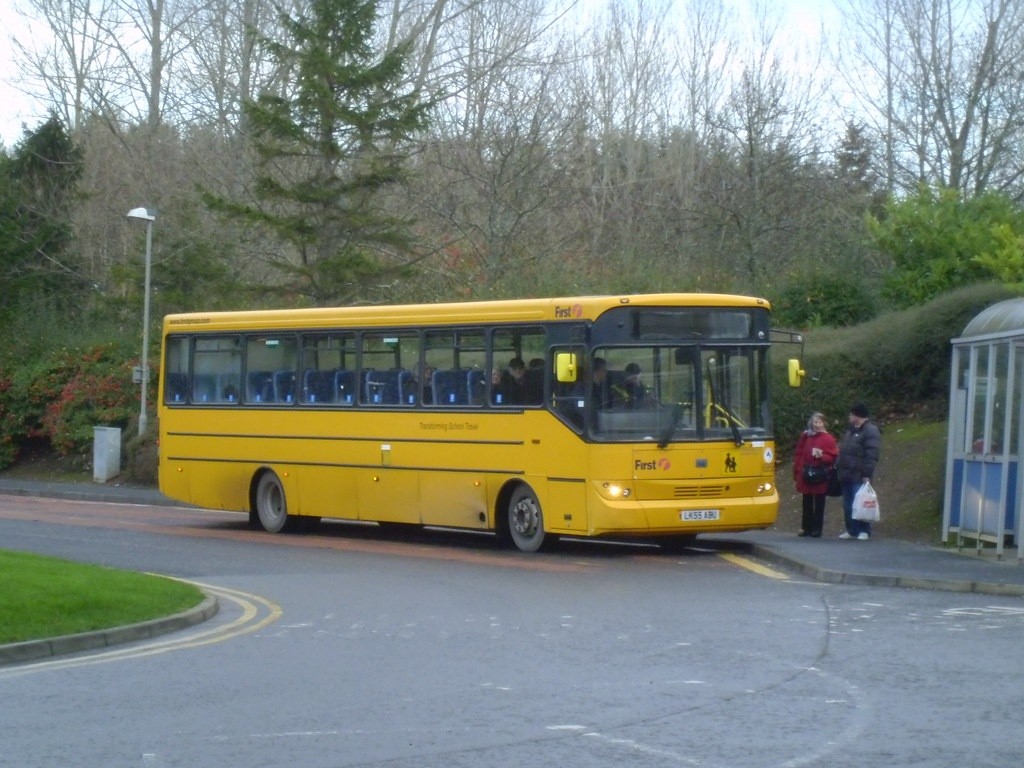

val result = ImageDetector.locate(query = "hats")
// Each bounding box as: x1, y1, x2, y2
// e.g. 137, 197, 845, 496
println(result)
509, 357, 524, 366
530, 358, 545, 366
852, 404, 869, 417
625, 363, 641, 374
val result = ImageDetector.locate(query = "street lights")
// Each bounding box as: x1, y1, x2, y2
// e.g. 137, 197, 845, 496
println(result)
127, 205, 156, 435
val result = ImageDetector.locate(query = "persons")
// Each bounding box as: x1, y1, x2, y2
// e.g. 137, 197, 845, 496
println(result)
609, 364, 651, 408
492, 358, 544, 405
569, 358, 612, 430
793, 412, 840, 537
835, 405, 881, 540
405, 361, 433, 405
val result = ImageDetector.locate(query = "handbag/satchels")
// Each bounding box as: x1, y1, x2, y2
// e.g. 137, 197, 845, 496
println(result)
803, 465, 826, 484
827, 469, 843, 496
852, 480, 879, 523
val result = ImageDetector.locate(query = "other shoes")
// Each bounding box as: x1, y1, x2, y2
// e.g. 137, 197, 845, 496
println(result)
811, 529, 819, 536
798, 528, 810, 536
857, 532, 870, 540
838, 531, 857, 540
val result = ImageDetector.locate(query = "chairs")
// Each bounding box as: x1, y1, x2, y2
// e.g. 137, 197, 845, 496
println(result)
608, 370, 626, 407
167, 366, 550, 407
554, 366, 583, 398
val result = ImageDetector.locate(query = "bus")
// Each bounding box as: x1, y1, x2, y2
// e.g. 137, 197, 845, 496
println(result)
156, 293, 807, 553
